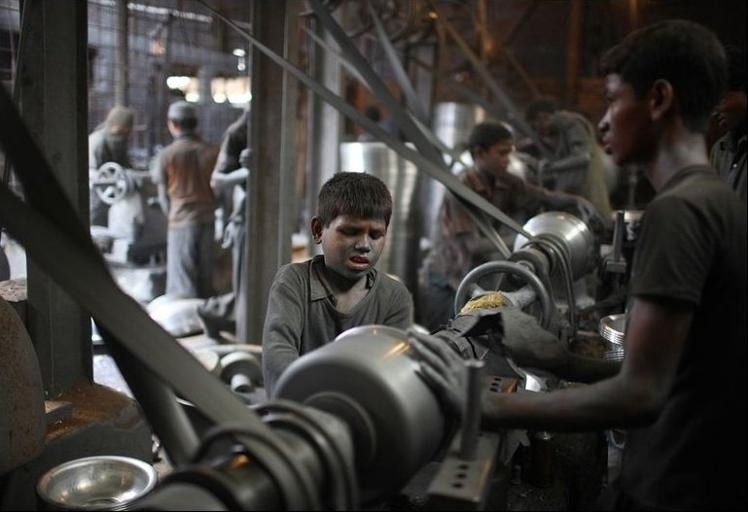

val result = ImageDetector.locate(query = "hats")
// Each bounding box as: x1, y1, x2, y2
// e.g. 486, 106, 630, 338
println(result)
167, 101, 199, 120
104, 107, 135, 129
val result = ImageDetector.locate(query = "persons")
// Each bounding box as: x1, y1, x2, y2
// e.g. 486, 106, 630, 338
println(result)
87, 105, 150, 228
403, 19, 748, 512
261, 171, 415, 403
153, 99, 223, 301
706, 40, 748, 202
208, 107, 250, 299
419, 119, 595, 336
514, 100, 595, 201
355, 104, 402, 143
